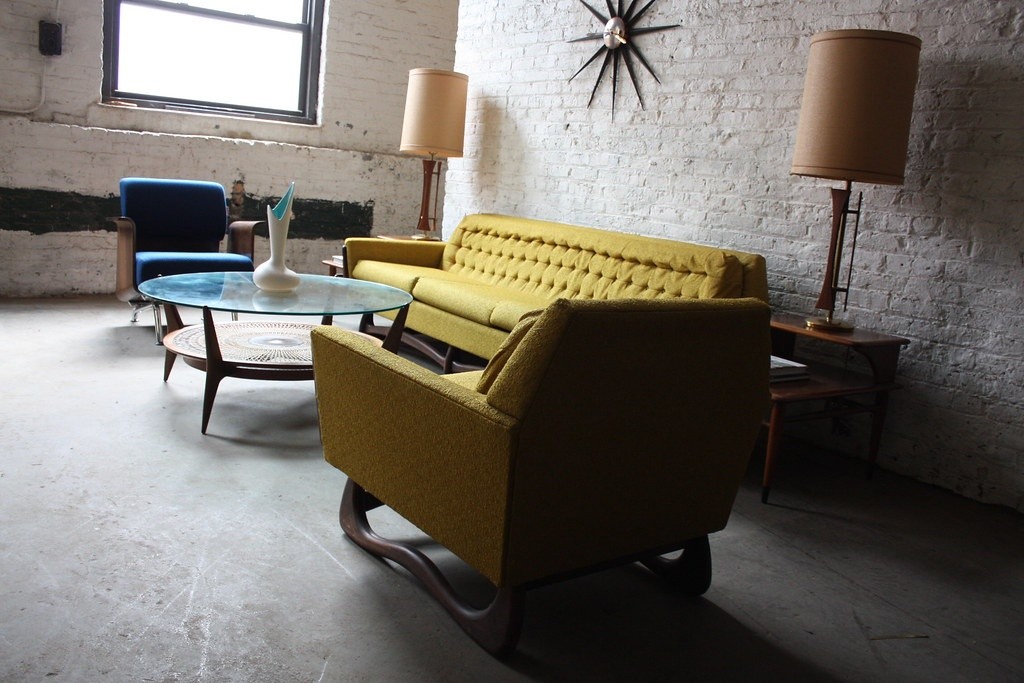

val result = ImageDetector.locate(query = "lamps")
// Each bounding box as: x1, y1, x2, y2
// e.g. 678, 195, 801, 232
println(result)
399, 67, 469, 241
789, 28, 921, 334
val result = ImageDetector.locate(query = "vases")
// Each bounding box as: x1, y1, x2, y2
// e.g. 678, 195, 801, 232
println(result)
251, 182, 302, 292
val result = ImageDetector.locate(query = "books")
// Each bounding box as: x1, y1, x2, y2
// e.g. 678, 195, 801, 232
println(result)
769, 374, 809, 384
771, 355, 808, 377
331, 255, 343, 265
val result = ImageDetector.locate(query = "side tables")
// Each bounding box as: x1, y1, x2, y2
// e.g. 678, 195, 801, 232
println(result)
763, 312, 911, 506
322, 235, 423, 277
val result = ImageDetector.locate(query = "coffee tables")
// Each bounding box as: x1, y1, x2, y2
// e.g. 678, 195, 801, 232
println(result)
137, 272, 413, 435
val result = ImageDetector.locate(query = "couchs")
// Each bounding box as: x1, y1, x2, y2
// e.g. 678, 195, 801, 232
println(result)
341, 214, 768, 376
105, 178, 266, 346
311, 296, 771, 654
